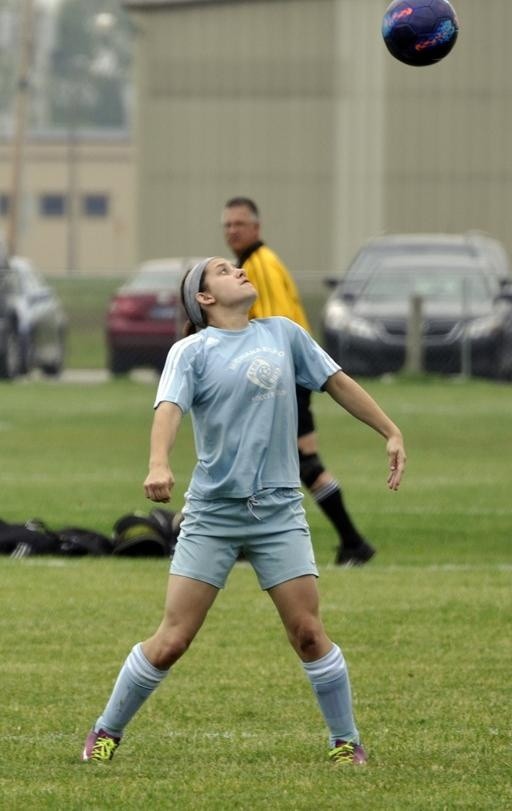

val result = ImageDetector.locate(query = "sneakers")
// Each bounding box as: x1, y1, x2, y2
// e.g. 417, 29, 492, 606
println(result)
83, 723, 122, 765
334, 539, 374, 566
331, 738, 368, 768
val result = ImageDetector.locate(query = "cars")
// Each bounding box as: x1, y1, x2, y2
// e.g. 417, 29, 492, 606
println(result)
0, 256, 68, 376
318, 229, 511, 387
106, 255, 200, 377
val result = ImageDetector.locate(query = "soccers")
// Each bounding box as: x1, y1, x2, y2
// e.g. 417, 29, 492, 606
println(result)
381, 1, 459, 66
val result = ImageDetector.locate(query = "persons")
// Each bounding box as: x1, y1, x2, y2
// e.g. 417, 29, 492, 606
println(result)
79, 257, 408, 765
221, 196, 376, 571
